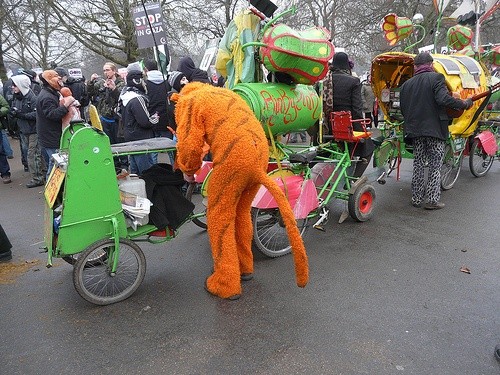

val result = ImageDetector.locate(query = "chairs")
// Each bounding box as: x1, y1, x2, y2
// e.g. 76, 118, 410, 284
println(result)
331, 112, 371, 159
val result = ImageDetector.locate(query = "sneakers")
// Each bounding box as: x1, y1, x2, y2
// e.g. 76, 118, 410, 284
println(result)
0, 173, 11, 184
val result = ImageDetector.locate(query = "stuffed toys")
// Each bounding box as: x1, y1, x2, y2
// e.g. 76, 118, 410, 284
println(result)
170, 82, 309, 299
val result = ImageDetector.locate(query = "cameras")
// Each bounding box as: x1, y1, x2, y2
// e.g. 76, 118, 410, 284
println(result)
98, 87, 107, 93
11, 107, 20, 117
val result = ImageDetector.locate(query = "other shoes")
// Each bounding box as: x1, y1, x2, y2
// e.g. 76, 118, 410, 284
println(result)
122, 165, 128, 172
425, 202, 445, 209
343, 180, 354, 190
204, 276, 240, 300
24, 165, 28, 171
211, 266, 252, 281
0, 250, 12, 261
26, 182, 43, 188
8, 156, 13, 159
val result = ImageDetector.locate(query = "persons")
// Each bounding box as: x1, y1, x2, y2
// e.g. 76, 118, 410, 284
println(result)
0, 57, 228, 188
323, 52, 376, 190
399, 52, 474, 209
361, 73, 378, 130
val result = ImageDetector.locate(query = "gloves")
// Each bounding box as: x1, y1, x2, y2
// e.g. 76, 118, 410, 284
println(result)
466, 98, 474, 111
9, 107, 25, 120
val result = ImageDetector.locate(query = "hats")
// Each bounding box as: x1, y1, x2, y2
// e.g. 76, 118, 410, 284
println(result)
126, 70, 145, 93
167, 71, 185, 89
332, 52, 349, 71
126, 64, 142, 72
414, 53, 433, 66
